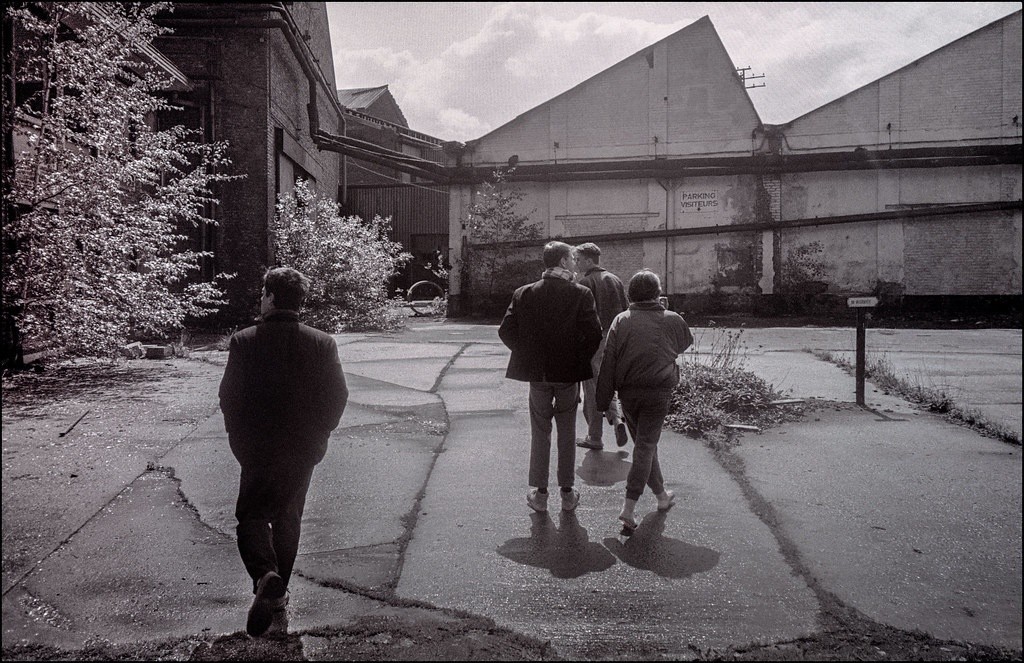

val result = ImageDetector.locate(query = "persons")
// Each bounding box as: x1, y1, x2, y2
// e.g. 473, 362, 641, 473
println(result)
572, 243, 630, 451
218, 268, 350, 638
498, 241, 603, 512
595, 268, 695, 530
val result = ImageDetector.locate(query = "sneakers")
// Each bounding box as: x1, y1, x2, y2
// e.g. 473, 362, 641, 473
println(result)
561, 487, 581, 511
527, 488, 549, 512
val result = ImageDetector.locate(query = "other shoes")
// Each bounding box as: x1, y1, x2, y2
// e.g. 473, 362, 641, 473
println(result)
577, 436, 604, 450
615, 422, 628, 447
273, 588, 290, 612
247, 572, 283, 638
618, 511, 637, 530
658, 489, 676, 511
